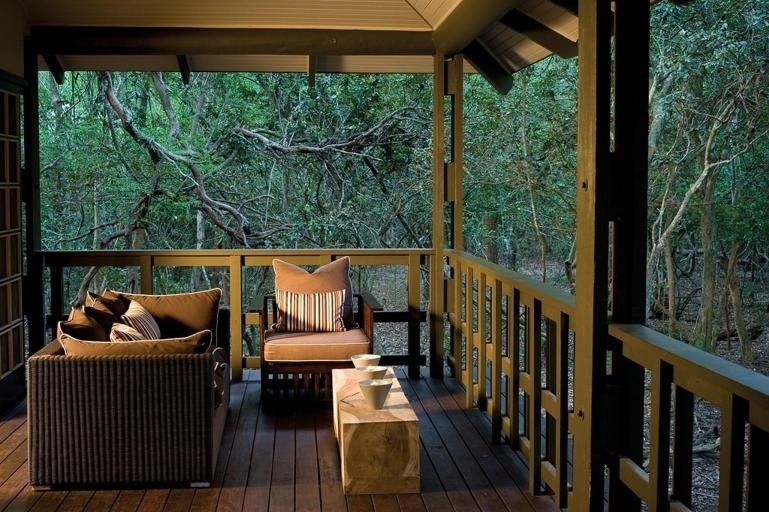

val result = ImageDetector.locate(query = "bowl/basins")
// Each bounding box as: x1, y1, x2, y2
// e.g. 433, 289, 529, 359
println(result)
353, 365, 388, 380
358, 378, 394, 410
351, 353, 381, 369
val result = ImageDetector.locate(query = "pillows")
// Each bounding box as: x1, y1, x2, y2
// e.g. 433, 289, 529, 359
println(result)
270, 257, 354, 333
60, 330, 213, 355
57, 307, 109, 344
118, 288, 221, 346
83, 297, 116, 325
112, 322, 149, 341
121, 300, 161, 340
84, 289, 118, 315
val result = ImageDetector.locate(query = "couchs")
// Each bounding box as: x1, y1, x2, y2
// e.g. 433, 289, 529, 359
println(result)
27, 287, 229, 492
260, 256, 383, 414
